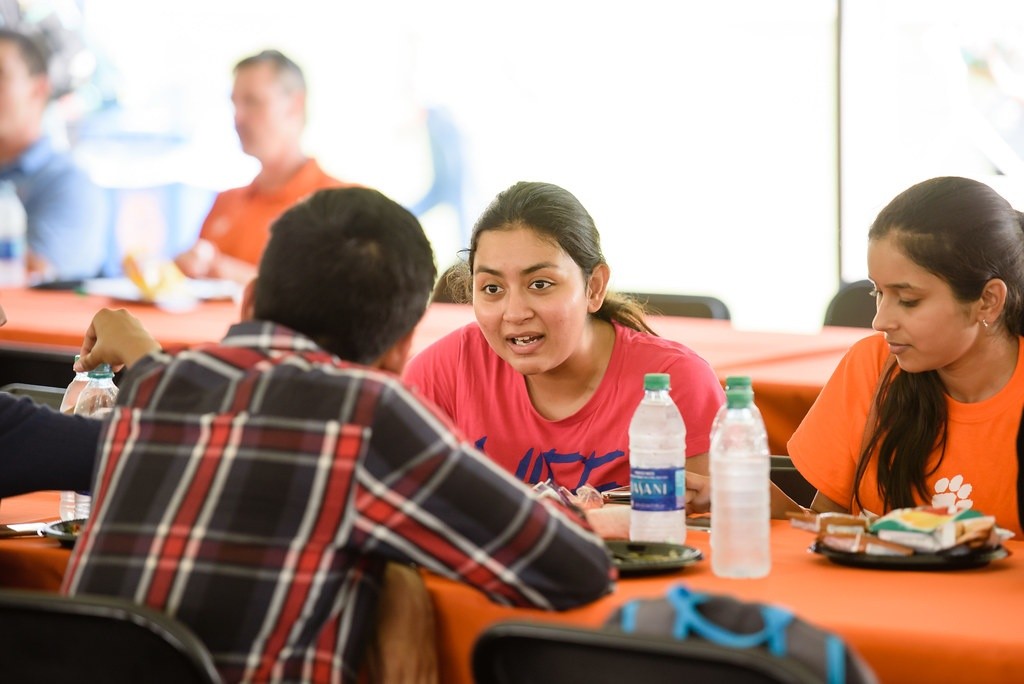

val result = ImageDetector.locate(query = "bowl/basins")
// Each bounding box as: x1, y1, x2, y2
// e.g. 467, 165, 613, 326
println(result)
584, 505, 630, 541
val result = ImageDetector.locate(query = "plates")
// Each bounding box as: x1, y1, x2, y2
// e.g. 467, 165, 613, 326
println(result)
79, 273, 235, 304
36, 518, 89, 549
808, 541, 1012, 570
607, 541, 703, 574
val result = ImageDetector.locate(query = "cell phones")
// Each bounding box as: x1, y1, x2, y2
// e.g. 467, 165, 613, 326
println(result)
601, 484, 631, 500
0, 522, 48, 538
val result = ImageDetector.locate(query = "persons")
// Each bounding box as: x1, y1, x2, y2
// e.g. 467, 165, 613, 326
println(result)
0, 287, 173, 500
684, 177, 1024, 536
177, 45, 352, 287
401, 179, 725, 502
0, 29, 106, 289
56, 188, 611, 684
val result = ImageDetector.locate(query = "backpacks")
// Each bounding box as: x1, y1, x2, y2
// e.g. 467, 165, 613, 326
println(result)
606, 586, 876, 684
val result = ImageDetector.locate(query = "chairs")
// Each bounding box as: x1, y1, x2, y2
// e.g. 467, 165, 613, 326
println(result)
0, 588, 224, 684
469, 620, 822, 683
820, 281, 881, 330
615, 291, 731, 320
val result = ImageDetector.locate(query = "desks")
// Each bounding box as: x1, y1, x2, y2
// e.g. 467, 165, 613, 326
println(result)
0, 281, 877, 456
0, 488, 1024, 684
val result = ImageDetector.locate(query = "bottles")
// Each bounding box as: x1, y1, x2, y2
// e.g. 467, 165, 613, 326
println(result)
628, 372, 688, 546
59, 353, 121, 521
0, 178, 28, 290
708, 376, 772, 580
